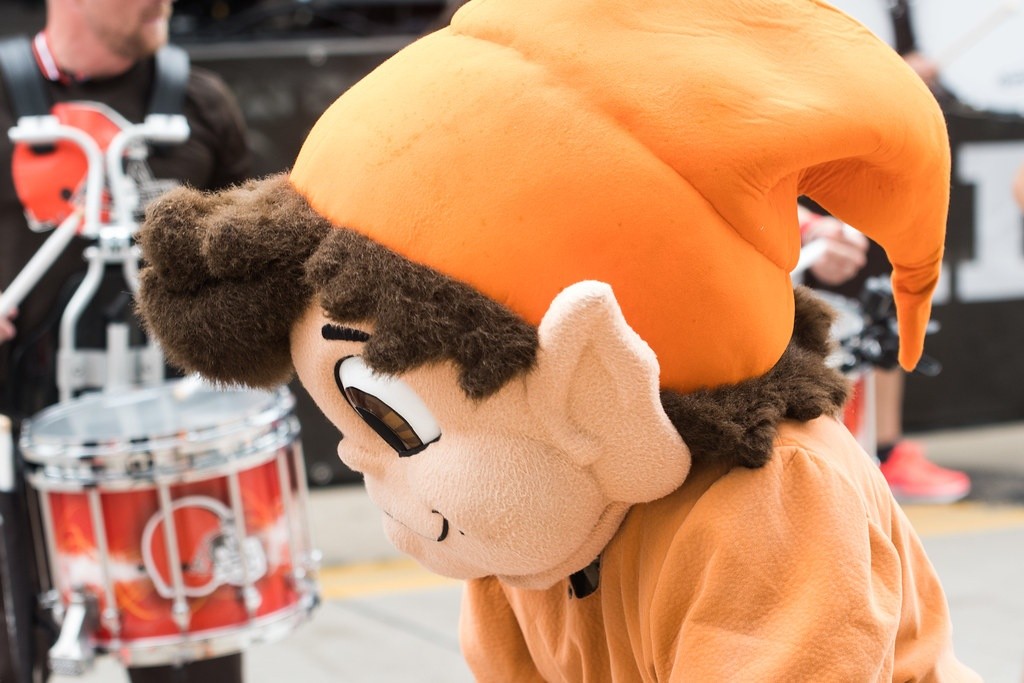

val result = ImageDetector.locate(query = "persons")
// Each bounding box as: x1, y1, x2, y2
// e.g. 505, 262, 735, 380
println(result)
787, 59, 973, 505
0, 0, 277, 683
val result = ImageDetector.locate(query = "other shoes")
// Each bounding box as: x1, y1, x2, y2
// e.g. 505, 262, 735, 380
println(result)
883, 443, 968, 504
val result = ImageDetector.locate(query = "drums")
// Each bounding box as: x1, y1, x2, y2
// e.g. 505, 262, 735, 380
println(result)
18, 373, 323, 669
810, 284, 883, 470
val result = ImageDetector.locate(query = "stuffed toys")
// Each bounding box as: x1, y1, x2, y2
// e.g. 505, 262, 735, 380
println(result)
133, 0, 982, 682
